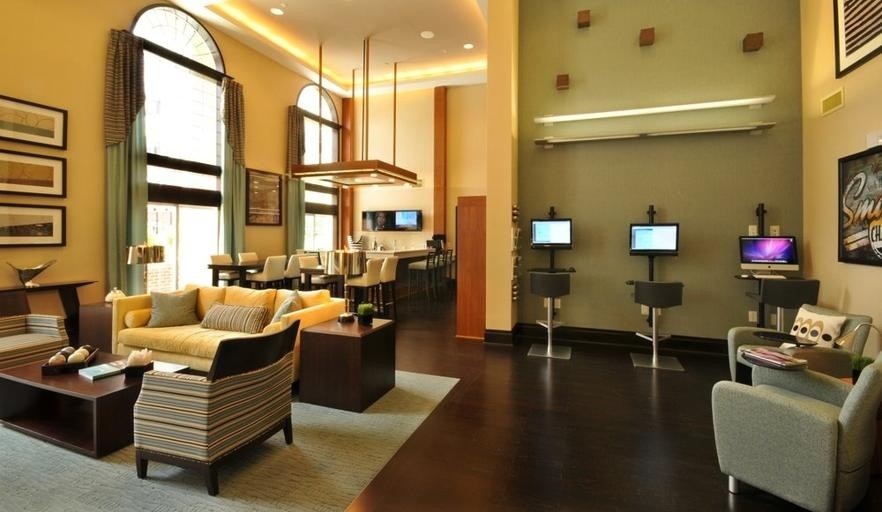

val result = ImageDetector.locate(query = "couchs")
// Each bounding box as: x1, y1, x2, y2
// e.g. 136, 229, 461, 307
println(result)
112, 283, 350, 388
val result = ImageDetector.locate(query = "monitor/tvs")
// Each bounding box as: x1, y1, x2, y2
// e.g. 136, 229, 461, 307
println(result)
630, 223, 681, 256
395, 210, 422, 230
739, 235, 802, 272
530, 218, 573, 250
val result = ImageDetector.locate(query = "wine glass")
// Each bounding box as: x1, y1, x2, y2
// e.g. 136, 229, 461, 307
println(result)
392, 239, 398, 251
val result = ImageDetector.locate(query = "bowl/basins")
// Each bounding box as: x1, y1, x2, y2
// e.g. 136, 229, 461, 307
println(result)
339, 314, 356, 323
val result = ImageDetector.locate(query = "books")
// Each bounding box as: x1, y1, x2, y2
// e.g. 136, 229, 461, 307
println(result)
740, 347, 809, 367
78, 358, 128, 381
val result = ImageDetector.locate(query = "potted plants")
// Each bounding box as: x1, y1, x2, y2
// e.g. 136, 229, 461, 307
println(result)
848, 354, 875, 384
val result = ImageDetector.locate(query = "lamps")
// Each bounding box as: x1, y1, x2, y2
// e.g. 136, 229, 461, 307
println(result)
126, 245, 165, 293
531, 93, 776, 128
292, 34, 421, 189
836, 321, 882, 352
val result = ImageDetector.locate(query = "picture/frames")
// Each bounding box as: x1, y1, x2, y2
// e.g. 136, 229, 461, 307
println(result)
832, 0, 882, 80
0, 201, 66, 247
0, 94, 68, 151
0, 149, 66, 198
245, 167, 282, 226
837, 143, 882, 270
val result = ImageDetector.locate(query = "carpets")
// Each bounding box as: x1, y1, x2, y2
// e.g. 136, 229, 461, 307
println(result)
0, 350, 461, 512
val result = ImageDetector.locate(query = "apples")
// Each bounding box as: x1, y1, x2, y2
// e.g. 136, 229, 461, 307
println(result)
49, 344, 92, 366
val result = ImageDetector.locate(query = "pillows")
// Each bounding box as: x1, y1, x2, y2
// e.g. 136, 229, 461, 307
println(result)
270, 288, 302, 323
201, 300, 269, 334
789, 306, 848, 352
146, 287, 201, 326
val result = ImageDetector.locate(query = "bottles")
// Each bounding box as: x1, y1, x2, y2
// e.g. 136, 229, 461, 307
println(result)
371, 237, 385, 251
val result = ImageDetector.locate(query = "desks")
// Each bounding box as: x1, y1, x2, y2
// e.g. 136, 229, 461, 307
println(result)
735, 270, 807, 327
0, 278, 99, 333
301, 313, 397, 417
752, 330, 820, 349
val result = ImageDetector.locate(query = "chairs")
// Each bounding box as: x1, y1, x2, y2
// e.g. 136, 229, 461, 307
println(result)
631, 280, 685, 373
528, 269, 575, 359
725, 298, 874, 384
210, 247, 455, 321
744, 275, 820, 336
0, 310, 72, 372
708, 352, 881, 511
133, 319, 300, 497
